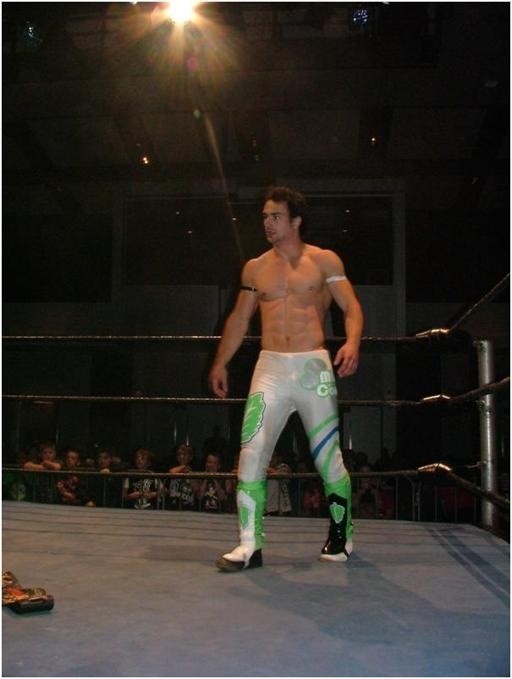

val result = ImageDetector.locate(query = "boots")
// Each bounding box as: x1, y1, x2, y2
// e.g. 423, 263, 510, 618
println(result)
319, 472, 353, 562
217, 481, 262, 570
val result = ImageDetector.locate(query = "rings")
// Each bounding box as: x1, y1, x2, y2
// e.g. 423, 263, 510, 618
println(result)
350, 359, 355, 362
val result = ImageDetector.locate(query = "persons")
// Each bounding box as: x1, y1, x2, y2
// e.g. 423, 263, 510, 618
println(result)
207, 186, 364, 572
